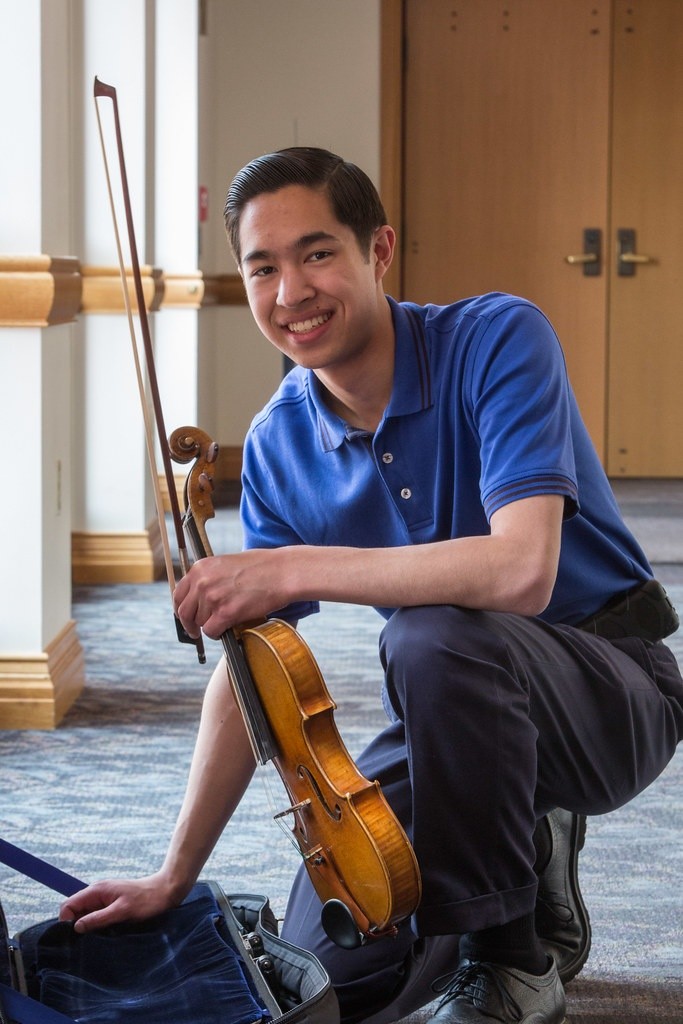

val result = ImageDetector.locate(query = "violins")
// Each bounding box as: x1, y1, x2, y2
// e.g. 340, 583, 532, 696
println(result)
165, 423, 426, 953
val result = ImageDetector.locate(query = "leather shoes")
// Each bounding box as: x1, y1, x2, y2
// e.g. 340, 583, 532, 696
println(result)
535, 805, 591, 988
391, 953, 566, 1024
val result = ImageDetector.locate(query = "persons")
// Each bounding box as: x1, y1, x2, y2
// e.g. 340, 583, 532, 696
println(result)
59, 146, 683, 1024
